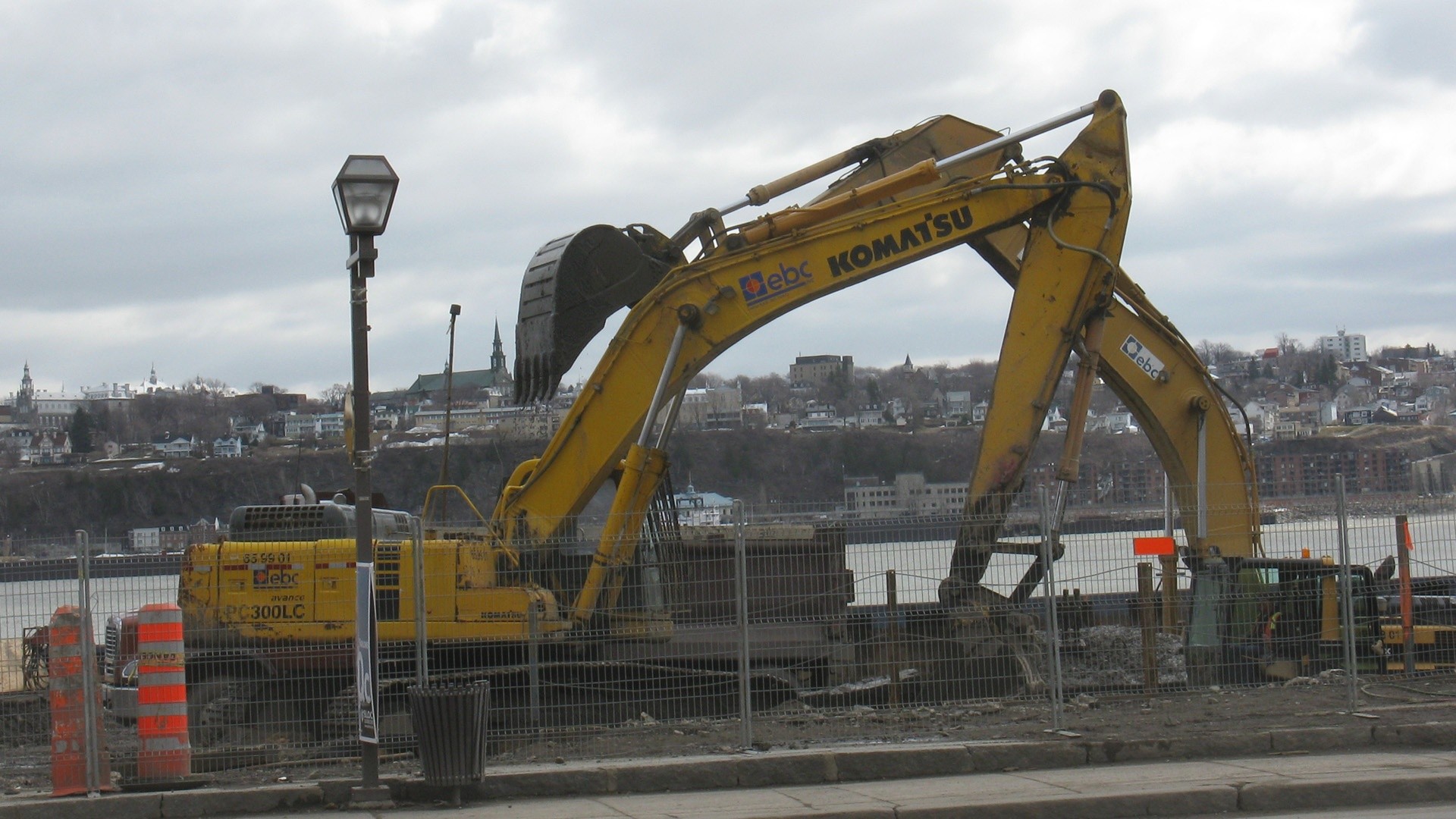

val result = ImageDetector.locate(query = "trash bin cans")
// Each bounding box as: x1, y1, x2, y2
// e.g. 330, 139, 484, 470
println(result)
406, 683, 490, 806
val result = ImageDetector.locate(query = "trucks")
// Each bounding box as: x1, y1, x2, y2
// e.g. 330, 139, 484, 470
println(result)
226, 482, 416, 542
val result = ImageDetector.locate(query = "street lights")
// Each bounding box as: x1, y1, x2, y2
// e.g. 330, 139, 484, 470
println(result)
327, 151, 403, 806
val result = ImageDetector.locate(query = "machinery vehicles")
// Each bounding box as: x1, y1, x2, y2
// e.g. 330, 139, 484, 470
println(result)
101, 89, 1126, 750
505, 110, 1401, 682
